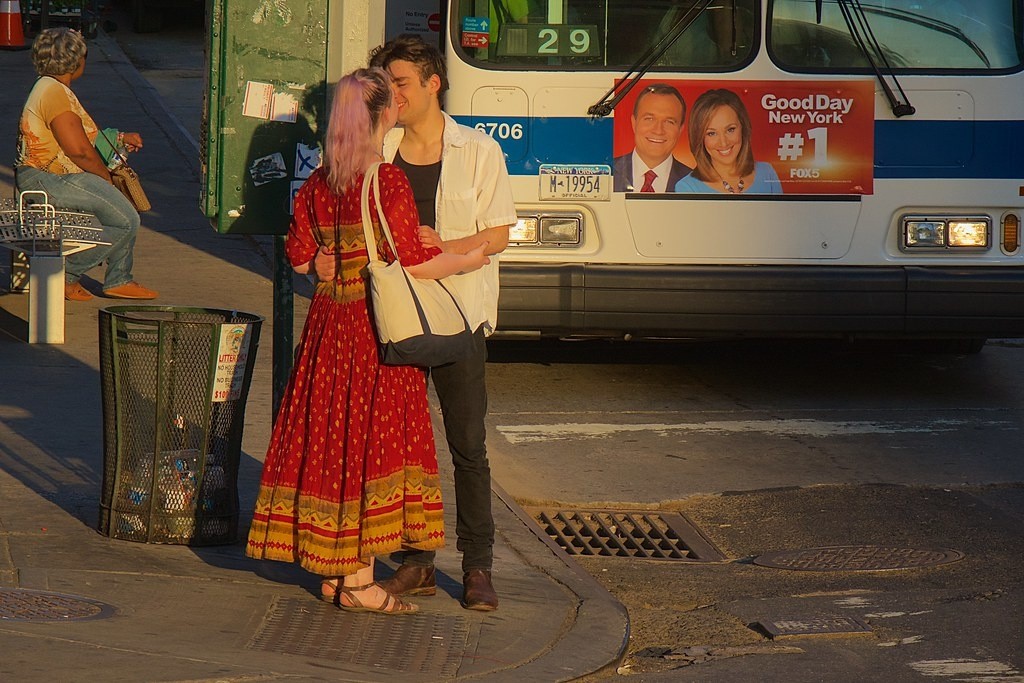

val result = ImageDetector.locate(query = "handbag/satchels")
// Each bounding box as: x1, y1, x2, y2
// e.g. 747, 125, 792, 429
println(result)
361, 162, 476, 366
111, 165, 151, 212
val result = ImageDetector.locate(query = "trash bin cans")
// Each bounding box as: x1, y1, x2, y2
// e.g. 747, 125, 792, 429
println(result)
97, 303, 266, 547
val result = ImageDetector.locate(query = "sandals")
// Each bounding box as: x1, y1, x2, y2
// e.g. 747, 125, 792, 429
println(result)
321, 578, 341, 604
339, 581, 420, 615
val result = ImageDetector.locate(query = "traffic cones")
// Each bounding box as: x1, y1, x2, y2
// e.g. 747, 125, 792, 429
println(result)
0, 0, 33, 51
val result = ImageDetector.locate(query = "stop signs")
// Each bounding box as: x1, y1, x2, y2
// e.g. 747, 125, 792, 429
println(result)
428, 13, 439, 31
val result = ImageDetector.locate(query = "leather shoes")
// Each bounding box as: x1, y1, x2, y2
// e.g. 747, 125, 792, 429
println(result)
460, 570, 498, 611
378, 556, 435, 596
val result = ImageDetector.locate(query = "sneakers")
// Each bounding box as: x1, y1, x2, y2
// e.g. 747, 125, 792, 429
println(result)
104, 280, 158, 299
64, 283, 94, 301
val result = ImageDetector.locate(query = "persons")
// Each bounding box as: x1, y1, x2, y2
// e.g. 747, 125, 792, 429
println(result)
13, 25, 161, 303
314, 32, 520, 612
242, 67, 451, 614
674, 89, 785, 194
614, 84, 694, 193
650, 0, 738, 69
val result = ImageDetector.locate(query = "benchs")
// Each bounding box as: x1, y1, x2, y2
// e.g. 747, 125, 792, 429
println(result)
0, 181, 112, 345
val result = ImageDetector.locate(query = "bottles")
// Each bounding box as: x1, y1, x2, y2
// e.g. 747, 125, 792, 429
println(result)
107, 142, 129, 172
126, 448, 224, 533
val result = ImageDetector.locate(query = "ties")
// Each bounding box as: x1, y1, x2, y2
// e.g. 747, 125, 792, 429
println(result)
641, 170, 657, 193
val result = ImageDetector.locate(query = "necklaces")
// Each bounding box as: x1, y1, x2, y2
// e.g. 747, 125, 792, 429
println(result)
709, 162, 746, 193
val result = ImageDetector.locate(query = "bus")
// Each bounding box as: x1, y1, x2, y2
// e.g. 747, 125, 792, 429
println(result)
441, 0, 1024, 358
